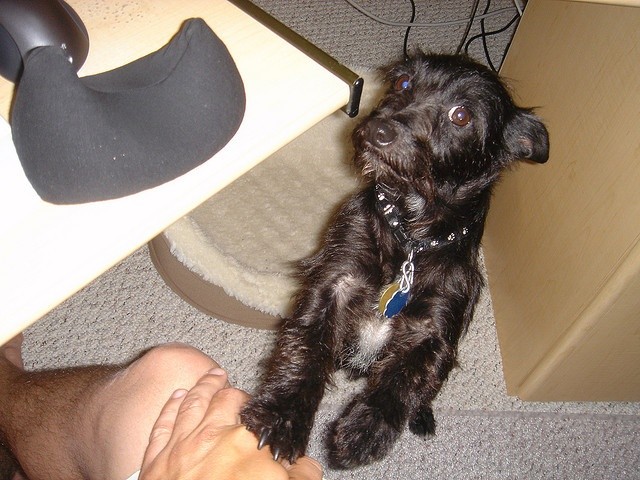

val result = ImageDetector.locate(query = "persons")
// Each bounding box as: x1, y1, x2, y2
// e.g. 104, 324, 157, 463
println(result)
0, 332, 326, 479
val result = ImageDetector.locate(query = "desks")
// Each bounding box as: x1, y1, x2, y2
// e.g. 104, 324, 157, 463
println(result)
1, 0, 362, 351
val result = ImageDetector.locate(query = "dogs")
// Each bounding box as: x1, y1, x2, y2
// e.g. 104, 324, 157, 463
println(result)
240, 49, 549, 469
